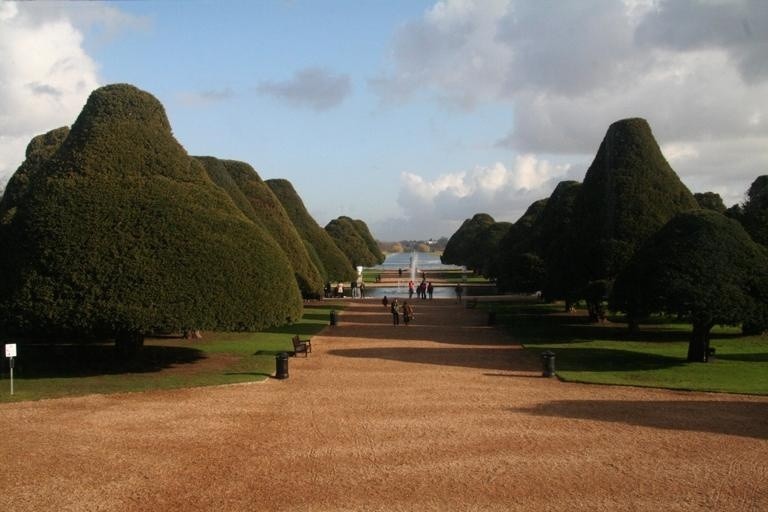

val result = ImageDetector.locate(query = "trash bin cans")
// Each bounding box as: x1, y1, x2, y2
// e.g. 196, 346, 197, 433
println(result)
488, 312, 496, 326
541, 350, 556, 377
329, 311, 337, 329
275, 352, 289, 379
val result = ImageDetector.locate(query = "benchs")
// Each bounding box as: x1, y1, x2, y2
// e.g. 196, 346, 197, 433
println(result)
292, 333, 312, 359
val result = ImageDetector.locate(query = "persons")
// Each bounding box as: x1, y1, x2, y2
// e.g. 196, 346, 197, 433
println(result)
323, 267, 464, 327
